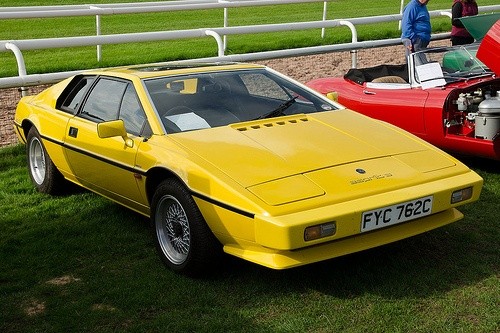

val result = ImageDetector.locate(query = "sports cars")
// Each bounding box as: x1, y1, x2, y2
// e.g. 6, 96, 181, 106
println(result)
305, 43, 500, 172
12, 59, 485, 275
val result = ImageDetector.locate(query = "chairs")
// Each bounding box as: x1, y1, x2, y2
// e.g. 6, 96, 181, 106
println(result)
200, 83, 231, 107
155, 92, 185, 117
372, 75, 406, 83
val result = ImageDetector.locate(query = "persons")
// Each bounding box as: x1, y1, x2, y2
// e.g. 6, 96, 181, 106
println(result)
401, 0, 432, 65
451, 0, 479, 46
